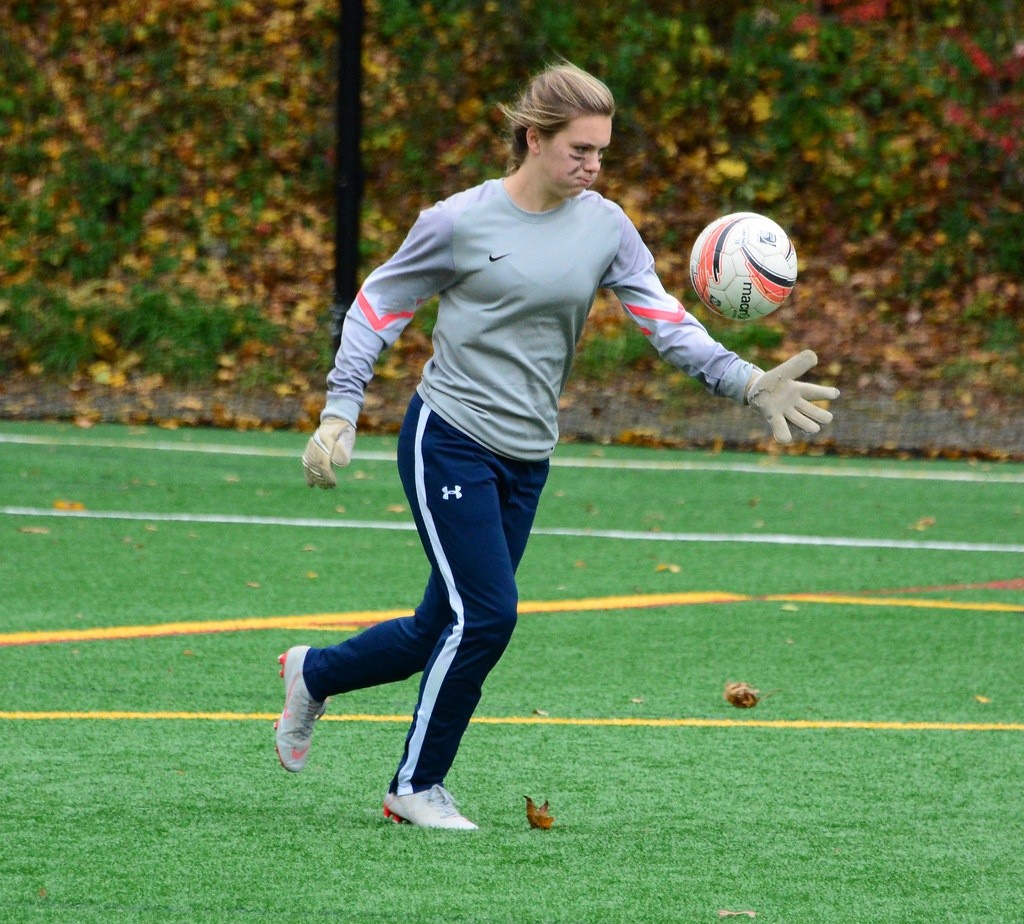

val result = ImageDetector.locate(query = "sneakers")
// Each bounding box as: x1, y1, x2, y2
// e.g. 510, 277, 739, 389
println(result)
273, 645, 325, 771
384, 785, 479, 830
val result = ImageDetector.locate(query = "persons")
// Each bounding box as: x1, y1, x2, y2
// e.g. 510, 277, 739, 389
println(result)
273, 60, 842, 831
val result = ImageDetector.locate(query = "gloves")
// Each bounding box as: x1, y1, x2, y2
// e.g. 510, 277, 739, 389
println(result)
745, 348, 840, 445
302, 416, 356, 490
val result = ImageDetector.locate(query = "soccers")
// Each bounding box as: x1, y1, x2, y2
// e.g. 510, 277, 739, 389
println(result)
689, 211, 798, 322
